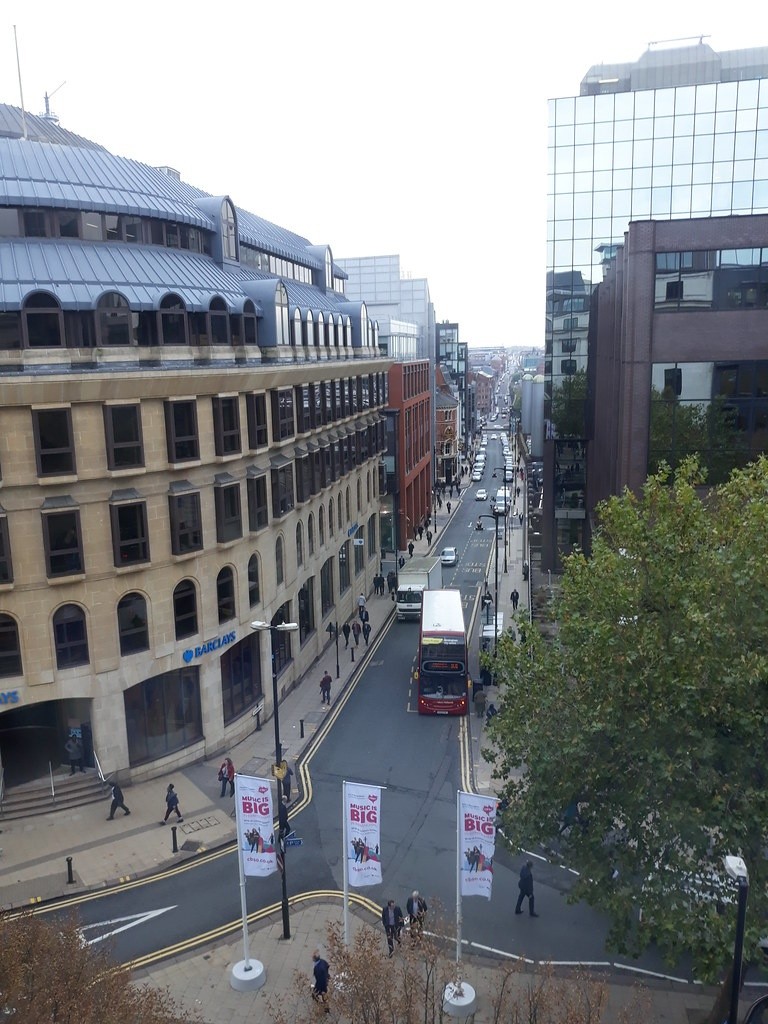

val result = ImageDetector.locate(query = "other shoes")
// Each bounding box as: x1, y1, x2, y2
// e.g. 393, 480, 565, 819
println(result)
177, 818, 183, 823
124, 811, 130, 815
106, 816, 114, 820
515, 911, 523, 914
160, 821, 166, 825
530, 913, 539, 917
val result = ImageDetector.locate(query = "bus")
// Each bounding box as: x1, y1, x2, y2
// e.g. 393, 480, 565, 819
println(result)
417, 588, 469, 717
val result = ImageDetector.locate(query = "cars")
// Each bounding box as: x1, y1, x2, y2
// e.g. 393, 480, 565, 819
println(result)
438, 546, 459, 567
471, 433, 488, 482
500, 432, 514, 482
339, 547, 346, 564
491, 433, 497, 440
493, 485, 510, 516
475, 488, 488, 501
481, 396, 510, 426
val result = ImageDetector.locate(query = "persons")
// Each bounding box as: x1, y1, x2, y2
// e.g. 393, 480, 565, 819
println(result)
398, 555, 406, 569
343, 622, 350, 649
283, 795, 290, 836
373, 573, 385, 595
282, 759, 294, 802
159, 784, 184, 825
484, 623, 528, 658
218, 758, 236, 798
516, 451, 525, 481
320, 671, 332, 705
408, 541, 414, 557
519, 512, 524, 525
461, 466, 472, 477
486, 591, 494, 605
510, 589, 519, 610
65, 734, 86, 776
473, 687, 497, 727
514, 859, 538, 917
106, 781, 130, 821
363, 622, 371, 645
522, 563, 529, 581
357, 593, 370, 621
406, 890, 427, 950
387, 569, 398, 593
516, 487, 521, 497
352, 620, 362, 646
435, 478, 462, 514
382, 901, 403, 958
311, 951, 330, 1013
413, 520, 432, 546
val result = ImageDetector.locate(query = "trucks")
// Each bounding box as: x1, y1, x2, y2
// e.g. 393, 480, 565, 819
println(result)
391, 556, 442, 622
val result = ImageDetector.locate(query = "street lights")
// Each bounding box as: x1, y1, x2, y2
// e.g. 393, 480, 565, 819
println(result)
249, 617, 298, 940
326, 621, 340, 679
722, 852, 748, 1024
474, 514, 498, 686
492, 464, 507, 573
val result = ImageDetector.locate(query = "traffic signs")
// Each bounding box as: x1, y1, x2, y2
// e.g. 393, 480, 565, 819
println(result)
286, 837, 305, 847
283, 829, 297, 845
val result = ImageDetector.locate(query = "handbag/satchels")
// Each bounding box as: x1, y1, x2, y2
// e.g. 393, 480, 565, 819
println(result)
217, 773, 222, 781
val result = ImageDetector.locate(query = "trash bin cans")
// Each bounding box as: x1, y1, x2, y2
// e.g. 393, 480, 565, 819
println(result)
473, 680, 484, 702
381, 548, 386, 559
480, 665, 491, 685
